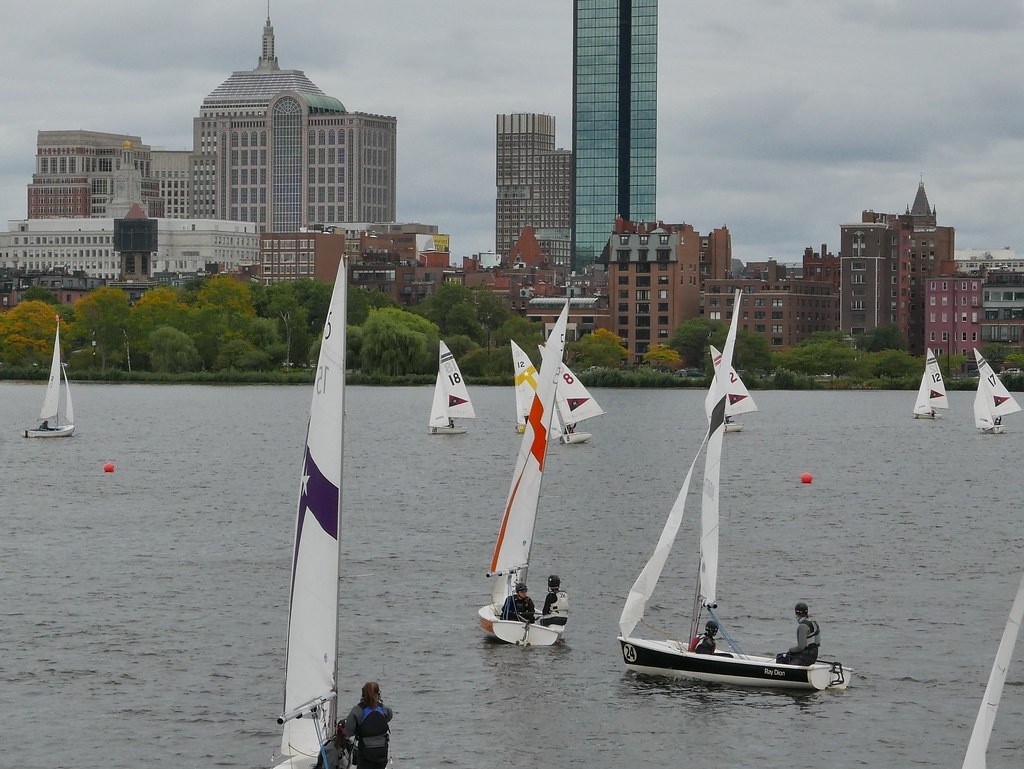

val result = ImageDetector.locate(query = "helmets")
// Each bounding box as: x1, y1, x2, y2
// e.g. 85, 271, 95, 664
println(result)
516, 583, 527, 593
548, 575, 560, 588
795, 603, 808, 613
706, 621, 719, 632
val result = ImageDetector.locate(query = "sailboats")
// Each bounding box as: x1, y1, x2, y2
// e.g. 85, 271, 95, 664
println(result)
22, 314, 76, 439
708, 343, 759, 433
912, 347, 949, 420
470, 295, 572, 650
427, 340, 476, 434
971, 347, 1022, 433
264, 255, 359, 769
537, 344, 606, 444
509, 338, 540, 433
618, 286, 856, 698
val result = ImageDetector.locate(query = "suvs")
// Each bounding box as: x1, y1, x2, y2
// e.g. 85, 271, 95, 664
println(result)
1000, 367, 1020, 376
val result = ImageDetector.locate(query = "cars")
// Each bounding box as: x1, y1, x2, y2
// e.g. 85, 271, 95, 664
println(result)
654, 366, 707, 378
280, 360, 318, 369
815, 373, 831, 377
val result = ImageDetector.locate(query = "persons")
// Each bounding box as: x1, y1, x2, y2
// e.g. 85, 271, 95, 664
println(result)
564, 424, 574, 434
499, 582, 535, 624
695, 620, 734, 657
540, 575, 568, 627
341, 682, 393, 769
778, 603, 821, 666
38, 421, 50, 431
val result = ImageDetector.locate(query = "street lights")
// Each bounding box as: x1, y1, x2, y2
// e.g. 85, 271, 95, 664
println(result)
92, 328, 96, 368
